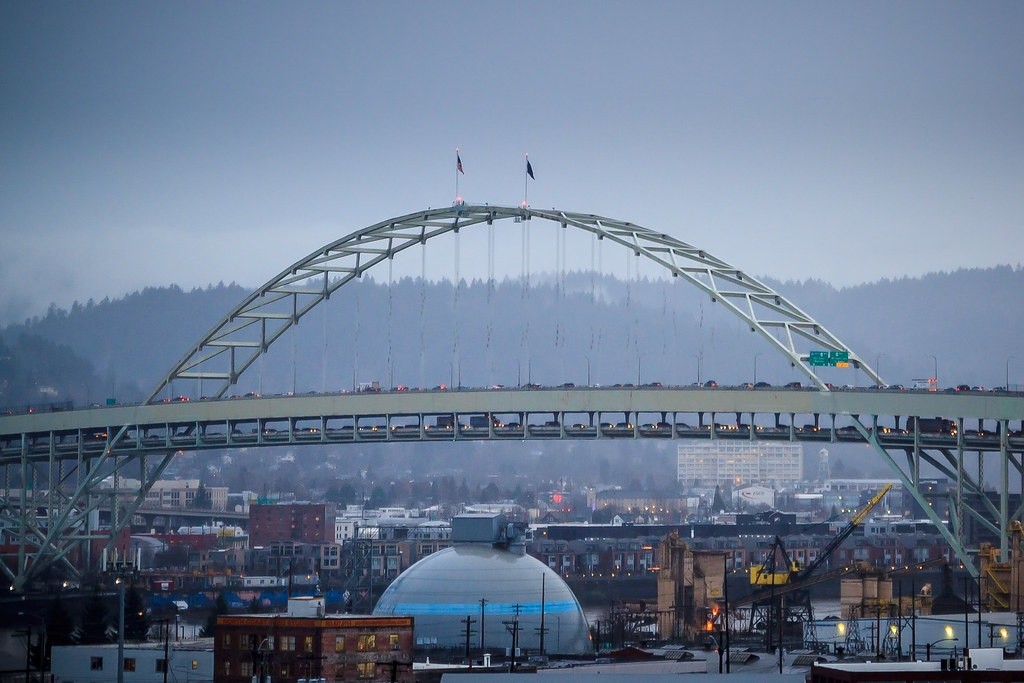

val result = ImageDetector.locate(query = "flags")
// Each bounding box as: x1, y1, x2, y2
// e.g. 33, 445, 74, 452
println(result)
457, 155, 464, 175
526, 160, 535, 180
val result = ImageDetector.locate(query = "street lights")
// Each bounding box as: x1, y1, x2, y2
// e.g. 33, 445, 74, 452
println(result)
926, 637, 960, 662
544, 612, 560, 652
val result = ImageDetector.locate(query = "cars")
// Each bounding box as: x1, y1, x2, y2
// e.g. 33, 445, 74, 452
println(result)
76, 377, 1024, 438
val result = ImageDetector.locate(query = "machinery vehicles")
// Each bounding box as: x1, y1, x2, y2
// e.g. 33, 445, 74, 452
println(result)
729, 483, 946, 610
